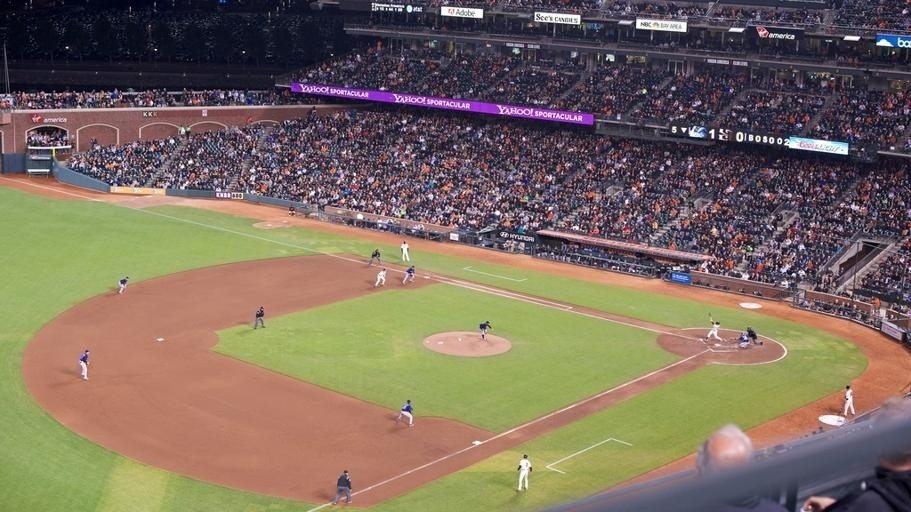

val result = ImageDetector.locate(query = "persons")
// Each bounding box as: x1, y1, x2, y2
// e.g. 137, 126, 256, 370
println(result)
398, 400, 415, 427
695, 423, 789, 512
843, 385, 856, 416
803, 396, 911, 512
332, 470, 353, 505
79, 350, 89, 380
0, 0, 911, 350
516, 454, 532, 491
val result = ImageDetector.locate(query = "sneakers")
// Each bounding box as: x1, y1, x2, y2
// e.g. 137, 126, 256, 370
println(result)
331, 500, 352, 505
840, 413, 855, 418
515, 487, 529, 492
374, 283, 384, 287
409, 423, 414, 428
81, 374, 89, 380
254, 326, 265, 329
